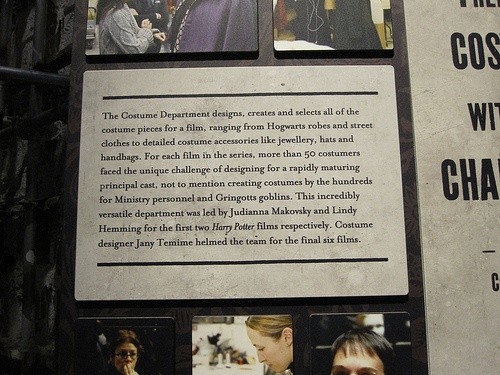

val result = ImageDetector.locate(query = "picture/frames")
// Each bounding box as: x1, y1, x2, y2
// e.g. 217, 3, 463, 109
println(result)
73, 316, 176, 375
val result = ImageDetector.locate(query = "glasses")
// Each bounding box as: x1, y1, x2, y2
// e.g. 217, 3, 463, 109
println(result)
112, 352, 138, 360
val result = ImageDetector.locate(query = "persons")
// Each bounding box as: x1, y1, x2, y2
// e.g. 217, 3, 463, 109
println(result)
96, 0, 383, 52
327, 313, 397, 375
245, 316, 294, 375
107, 330, 140, 374
